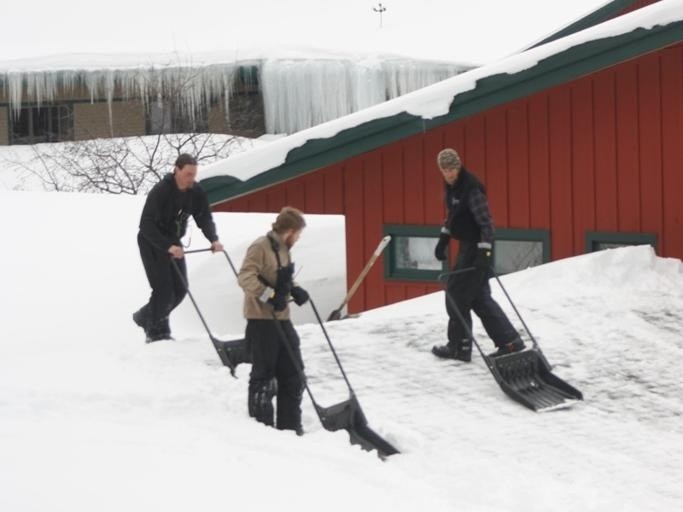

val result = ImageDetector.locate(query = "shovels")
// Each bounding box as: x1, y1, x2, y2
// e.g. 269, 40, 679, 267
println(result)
170, 248, 254, 378
328, 235, 391, 322
439, 266, 583, 413
271, 298, 400, 461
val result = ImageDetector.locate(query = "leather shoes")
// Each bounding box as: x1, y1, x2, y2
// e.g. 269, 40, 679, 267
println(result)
488, 339, 526, 356
431, 344, 472, 363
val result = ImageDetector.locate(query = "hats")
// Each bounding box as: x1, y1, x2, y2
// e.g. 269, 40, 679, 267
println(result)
437, 148, 461, 169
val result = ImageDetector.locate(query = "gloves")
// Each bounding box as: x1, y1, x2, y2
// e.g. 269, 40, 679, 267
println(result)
473, 242, 492, 270
434, 227, 450, 261
291, 282, 309, 306
259, 286, 287, 311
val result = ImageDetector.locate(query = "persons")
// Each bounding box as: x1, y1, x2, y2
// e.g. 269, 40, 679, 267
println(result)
237, 206, 309, 437
132, 153, 224, 346
432, 148, 526, 363
